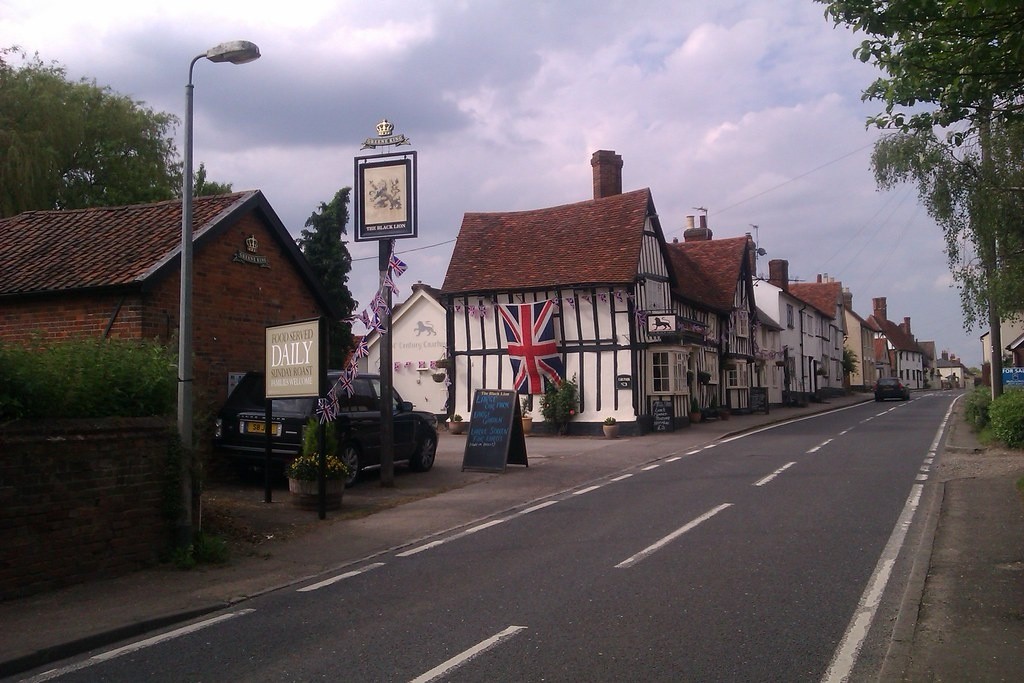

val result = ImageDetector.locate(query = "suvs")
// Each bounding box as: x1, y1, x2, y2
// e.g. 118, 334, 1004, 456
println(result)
216, 366, 438, 488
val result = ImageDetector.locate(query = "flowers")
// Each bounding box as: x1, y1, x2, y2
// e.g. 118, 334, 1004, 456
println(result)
291, 452, 351, 481
568, 410, 575, 416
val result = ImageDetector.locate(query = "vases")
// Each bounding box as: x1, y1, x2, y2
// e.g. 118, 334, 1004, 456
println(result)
288, 477, 346, 511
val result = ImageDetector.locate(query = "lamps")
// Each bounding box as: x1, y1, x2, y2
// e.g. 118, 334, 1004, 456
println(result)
206, 40, 263, 66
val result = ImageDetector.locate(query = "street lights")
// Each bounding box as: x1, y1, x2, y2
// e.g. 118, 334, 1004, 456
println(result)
176, 39, 265, 551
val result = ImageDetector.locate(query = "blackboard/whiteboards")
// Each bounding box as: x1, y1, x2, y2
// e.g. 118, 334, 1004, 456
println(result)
749, 387, 769, 413
462, 390, 529, 470
652, 400, 674, 432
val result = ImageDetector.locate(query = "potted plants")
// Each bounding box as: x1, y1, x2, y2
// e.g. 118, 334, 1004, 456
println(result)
719, 407, 730, 420
686, 369, 694, 385
435, 353, 451, 369
519, 397, 533, 437
774, 359, 833, 409
697, 370, 712, 386
603, 417, 620, 440
688, 396, 702, 424
431, 372, 446, 383
705, 394, 719, 420
636, 414, 655, 436
448, 415, 467, 435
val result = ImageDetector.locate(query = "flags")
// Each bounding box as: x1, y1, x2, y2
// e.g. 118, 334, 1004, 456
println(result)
497, 298, 566, 395
454, 290, 625, 318
315, 254, 408, 422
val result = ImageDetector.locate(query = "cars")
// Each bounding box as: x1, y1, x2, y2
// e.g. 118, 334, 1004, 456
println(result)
874, 378, 911, 400
